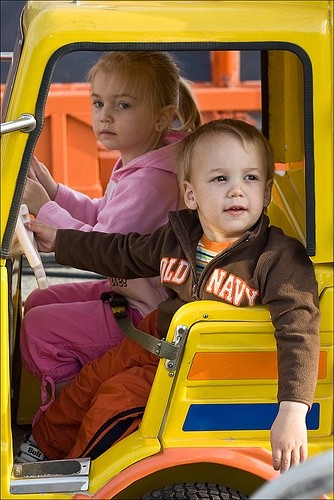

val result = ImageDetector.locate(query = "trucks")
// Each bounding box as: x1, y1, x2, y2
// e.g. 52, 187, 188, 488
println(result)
2, 0, 334, 500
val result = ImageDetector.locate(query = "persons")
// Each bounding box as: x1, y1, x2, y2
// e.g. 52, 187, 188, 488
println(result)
13, 117, 322, 476
19, 49, 203, 397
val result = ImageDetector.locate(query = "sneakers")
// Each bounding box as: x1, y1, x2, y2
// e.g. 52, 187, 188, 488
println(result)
12, 436, 46, 464
